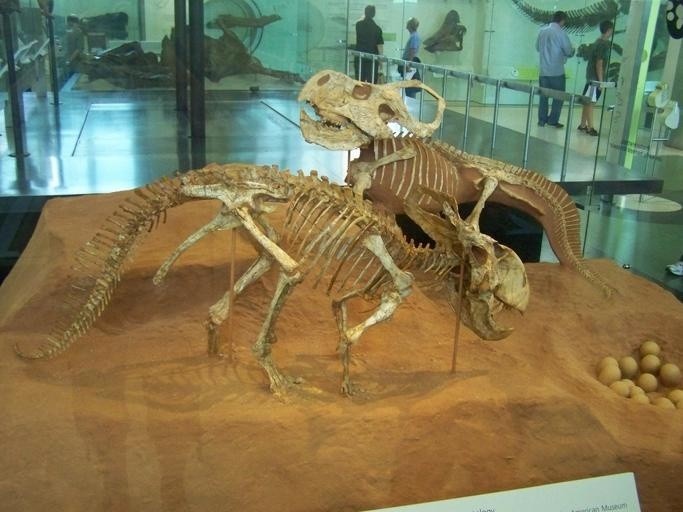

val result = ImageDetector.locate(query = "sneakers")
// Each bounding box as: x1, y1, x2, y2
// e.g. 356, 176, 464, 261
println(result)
578, 124, 598, 137
539, 120, 563, 128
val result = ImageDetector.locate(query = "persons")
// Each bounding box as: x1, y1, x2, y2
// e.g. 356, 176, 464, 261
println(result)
353, 5, 382, 84
65, 14, 84, 77
397, 17, 422, 100
663, 254, 683, 278
576, 21, 613, 136
533, 10, 575, 130
